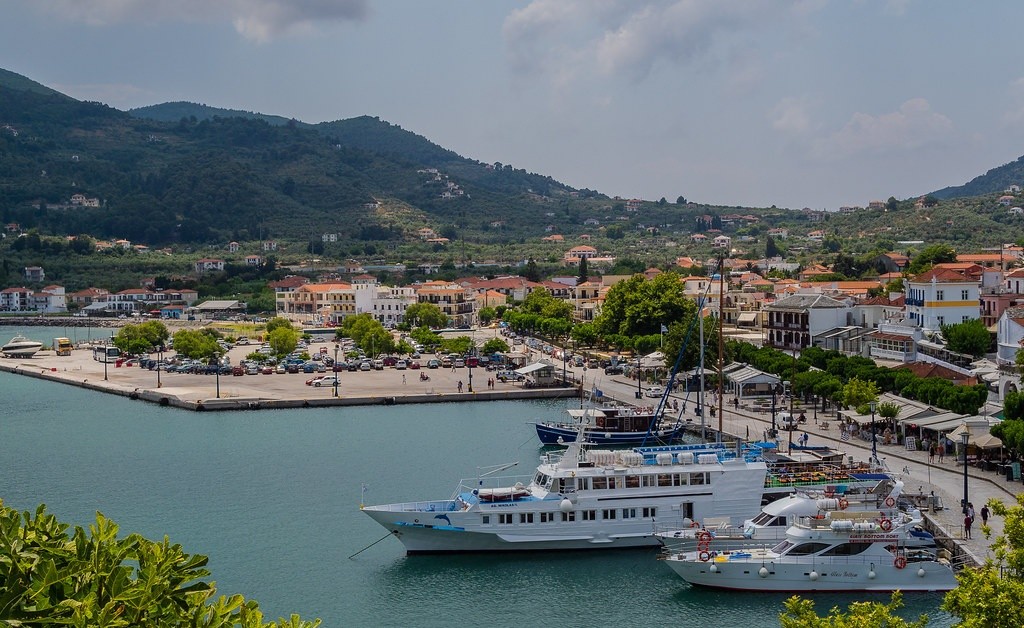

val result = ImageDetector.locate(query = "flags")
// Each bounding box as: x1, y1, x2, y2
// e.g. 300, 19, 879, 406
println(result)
662, 326, 668, 331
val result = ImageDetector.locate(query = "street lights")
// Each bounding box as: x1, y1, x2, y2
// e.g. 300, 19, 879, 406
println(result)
813, 395, 819, 418
466, 345, 474, 392
768, 381, 780, 430
562, 337, 568, 387
958, 430, 974, 513
695, 366, 701, 416
867, 400, 879, 458
333, 344, 342, 397
636, 355, 643, 399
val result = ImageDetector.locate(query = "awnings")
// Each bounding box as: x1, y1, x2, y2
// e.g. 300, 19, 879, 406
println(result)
837, 410, 893, 425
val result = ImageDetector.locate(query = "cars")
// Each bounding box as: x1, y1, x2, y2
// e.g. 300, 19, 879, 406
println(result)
501, 330, 570, 361
458, 324, 472, 329
306, 376, 325, 386
588, 358, 599, 369
124, 337, 335, 377
571, 356, 584, 367
645, 386, 663, 398
301, 320, 337, 329
488, 322, 500, 329
336, 332, 490, 369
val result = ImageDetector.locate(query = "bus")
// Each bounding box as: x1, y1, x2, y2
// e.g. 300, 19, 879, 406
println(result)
93, 345, 120, 363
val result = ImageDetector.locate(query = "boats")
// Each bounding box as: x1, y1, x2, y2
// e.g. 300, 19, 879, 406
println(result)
524, 375, 691, 450
356, 372, 766, 552
1, 335, 44, 359
653, 343, 961, 595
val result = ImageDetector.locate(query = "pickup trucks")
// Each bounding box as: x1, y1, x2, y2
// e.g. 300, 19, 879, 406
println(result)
496, 372, 525, 381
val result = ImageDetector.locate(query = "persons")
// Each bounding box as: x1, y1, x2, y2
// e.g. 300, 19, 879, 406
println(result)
798, 434, 803, 446
450, 360, 455, 372
849, 424, 854, 438
964, 513, 971, 539
804, 432, 808, 447
798, 413, 805, 422
938, 445, 944, 464
781, 394, 785, 403
403, 374, 406, 384
420, 372, 428, 381
968, 504, 974, 526
929, 444, 934, 464
671, 399, 679, 411
488, 378, 494, 390
839, 421, 845, 436
682, 402, 686, 413
457, 381, 463, 393
980, 505, 991, 526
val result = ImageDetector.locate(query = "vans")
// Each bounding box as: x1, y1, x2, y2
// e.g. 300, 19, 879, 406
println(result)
777, 413, 798, 431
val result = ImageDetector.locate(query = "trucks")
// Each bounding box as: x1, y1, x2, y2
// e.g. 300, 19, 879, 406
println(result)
53, 338, 73, 356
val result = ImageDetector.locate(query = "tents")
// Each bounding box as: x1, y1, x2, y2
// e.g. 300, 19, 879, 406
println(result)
974, 435, 1003, 462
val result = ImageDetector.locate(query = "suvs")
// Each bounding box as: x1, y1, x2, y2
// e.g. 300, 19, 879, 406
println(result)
311, 375, 342, 387
234, 338, 250, 346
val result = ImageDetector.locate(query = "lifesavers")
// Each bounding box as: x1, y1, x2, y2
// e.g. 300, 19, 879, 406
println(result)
599, 418, 604, 425
894, 556, 906, 569
635, 406, 642, 415
876, 512, 886, 522
690, 522, 700, 528
698, 532, 711, 561
880, 519, 891, 531
840, 499, 849, 510
648, 407, 653, 414
885, 497, 895, 507
